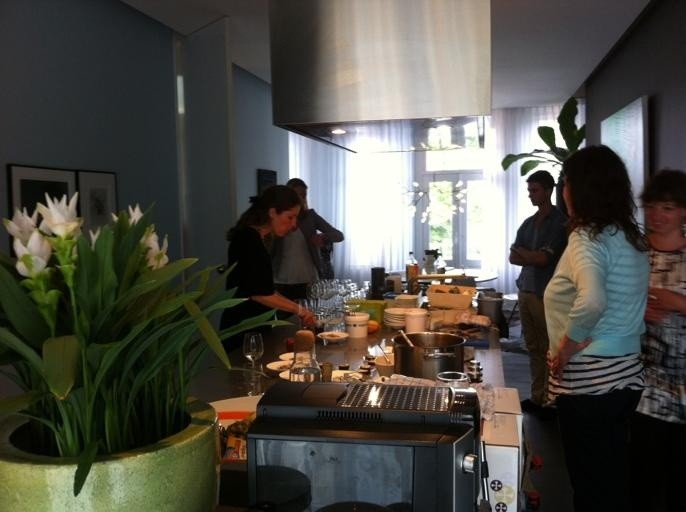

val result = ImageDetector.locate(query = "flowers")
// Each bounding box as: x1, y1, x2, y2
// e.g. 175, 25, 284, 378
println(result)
0, 191, 292, 498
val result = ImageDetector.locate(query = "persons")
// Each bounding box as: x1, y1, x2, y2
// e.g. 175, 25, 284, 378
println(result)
509, 171, 572, 413
216, 184, 316, 372
638, 167, 685, 510
541, 144, 654, 510
266, 178, 344, 319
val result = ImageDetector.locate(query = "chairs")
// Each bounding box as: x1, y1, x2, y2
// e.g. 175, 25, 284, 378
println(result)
502, 293, 523, 338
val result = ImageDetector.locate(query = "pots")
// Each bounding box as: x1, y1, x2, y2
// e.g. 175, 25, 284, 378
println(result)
391, 331, 466, 382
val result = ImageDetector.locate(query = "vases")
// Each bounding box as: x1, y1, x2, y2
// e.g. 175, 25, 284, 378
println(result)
0, 397, 221, 512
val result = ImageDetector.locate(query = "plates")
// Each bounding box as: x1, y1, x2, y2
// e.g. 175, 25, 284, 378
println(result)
384, 306, 408, 331
394, 293, 419, 311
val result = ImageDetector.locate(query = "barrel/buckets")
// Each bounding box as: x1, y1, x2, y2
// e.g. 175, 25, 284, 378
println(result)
344, 311, 370, 338
406, 251, 419, 284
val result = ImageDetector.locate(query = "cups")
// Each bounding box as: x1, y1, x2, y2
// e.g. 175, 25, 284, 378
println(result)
477, 292, 504, 323
403, 308, 429, 333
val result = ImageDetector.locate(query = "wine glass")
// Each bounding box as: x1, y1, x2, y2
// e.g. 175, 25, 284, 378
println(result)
241, 330, 264, 397
293, 275, 371, 346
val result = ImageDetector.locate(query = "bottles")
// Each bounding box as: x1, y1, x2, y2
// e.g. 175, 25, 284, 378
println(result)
468, 358, 484, 383
405, 251, 419, 295
420, 258, 428, 275
433, 253, 446, 284
424, 249, 438, 274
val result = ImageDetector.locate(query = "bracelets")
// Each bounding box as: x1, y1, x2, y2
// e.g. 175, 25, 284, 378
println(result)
297, 304, 303, 317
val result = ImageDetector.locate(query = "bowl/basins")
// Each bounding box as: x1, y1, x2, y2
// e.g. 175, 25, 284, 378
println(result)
434, 370, 471, 388
266, 345, 395, 385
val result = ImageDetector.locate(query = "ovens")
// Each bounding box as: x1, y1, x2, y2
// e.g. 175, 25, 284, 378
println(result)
244, 382, 492, 512
